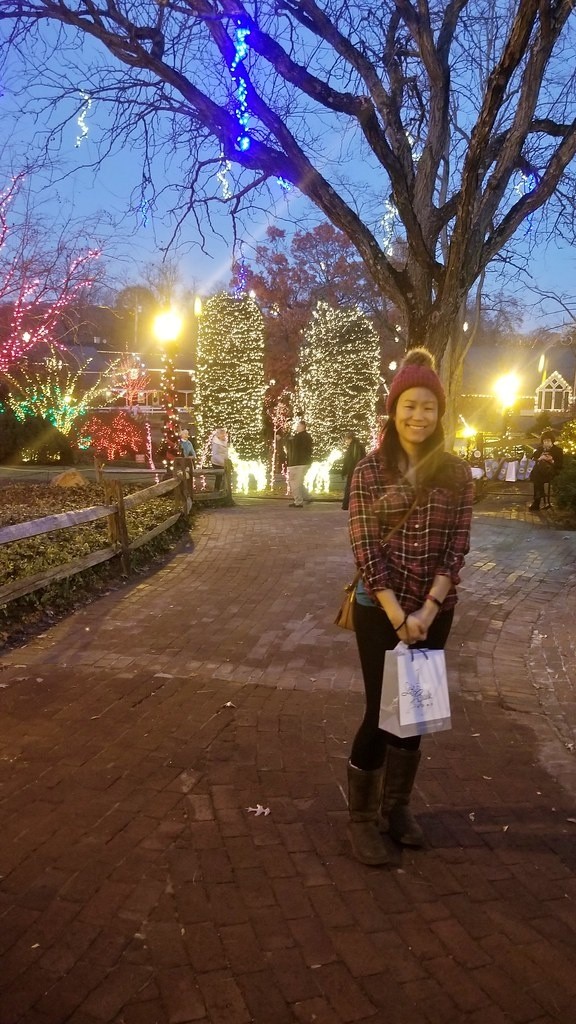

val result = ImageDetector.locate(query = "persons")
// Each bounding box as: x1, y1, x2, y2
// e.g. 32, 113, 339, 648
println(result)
180, 419, 562, 512
344, 347, 475, 864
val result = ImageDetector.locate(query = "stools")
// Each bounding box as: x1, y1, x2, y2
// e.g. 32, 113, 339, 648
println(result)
540, 481, 554, 509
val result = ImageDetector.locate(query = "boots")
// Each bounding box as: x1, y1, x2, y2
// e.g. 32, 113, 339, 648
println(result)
347, 757, 391, 865
380, 745, 423, 846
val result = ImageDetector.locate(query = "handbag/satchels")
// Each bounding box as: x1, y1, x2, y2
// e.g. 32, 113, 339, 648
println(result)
335, 580, 359, 632
377, 615, 453, 738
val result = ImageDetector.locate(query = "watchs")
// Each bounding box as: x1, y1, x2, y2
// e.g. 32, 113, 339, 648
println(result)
426, 594, 443, 611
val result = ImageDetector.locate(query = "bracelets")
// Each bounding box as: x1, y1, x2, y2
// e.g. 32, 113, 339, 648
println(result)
395, 613, 409, 632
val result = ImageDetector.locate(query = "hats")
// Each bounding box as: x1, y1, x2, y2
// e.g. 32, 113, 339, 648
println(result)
386, 346, 445, 422
